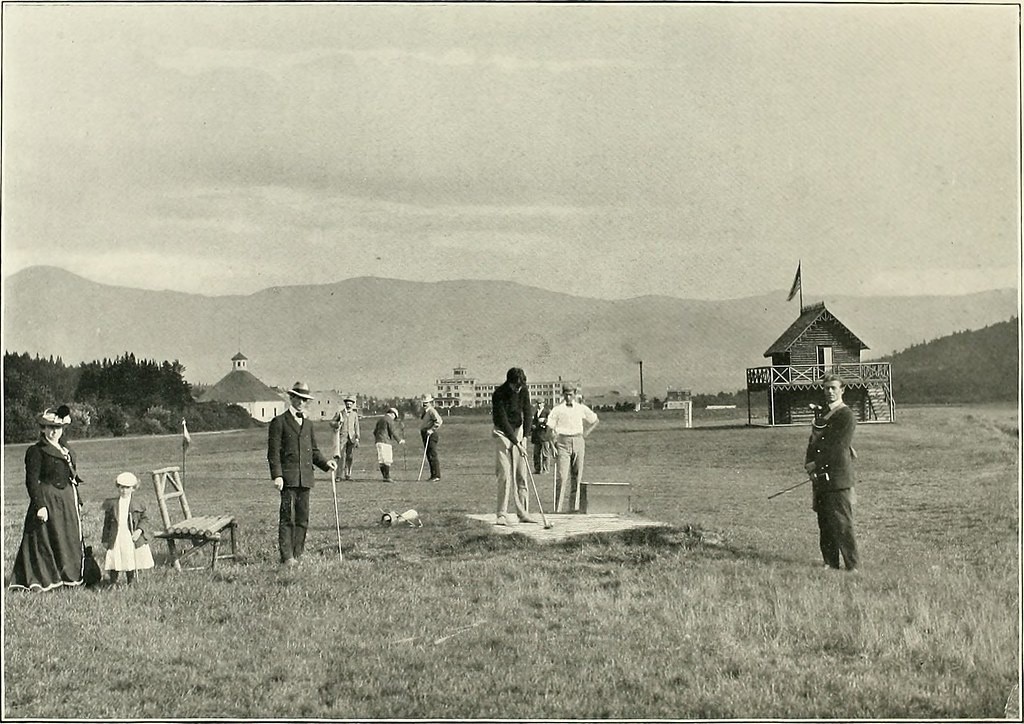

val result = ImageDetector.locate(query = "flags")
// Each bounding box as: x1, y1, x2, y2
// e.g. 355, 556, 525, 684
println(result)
786, 265, 800, 301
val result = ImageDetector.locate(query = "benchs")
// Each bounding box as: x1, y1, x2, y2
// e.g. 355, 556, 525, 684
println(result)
151, 466, 239, 572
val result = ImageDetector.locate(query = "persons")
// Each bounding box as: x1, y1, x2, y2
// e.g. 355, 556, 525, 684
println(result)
330, 396, 361, 481
489, 366, 538, 524
530, 395, 551, 476
802, 375, 862, 576
99, 472, 154, 584
5, 405, 86, 593
267, 382, 338, 563
418, 393, 443, 482
547, 380, 599, 513
374, 408, 406, 484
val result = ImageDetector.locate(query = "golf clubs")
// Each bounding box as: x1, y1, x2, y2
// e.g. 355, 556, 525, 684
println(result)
522, 452, 552, 530
418, 435, 431, 482
328, 466, 343, 566
768, 473, 831, 500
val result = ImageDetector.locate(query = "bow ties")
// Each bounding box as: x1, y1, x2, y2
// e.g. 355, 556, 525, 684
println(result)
296, 412, 303, 417
566, 403, 573, 406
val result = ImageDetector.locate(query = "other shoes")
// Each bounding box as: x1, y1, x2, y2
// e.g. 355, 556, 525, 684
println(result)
498, 516, 506, 526
336, 477, 340, 482
521, 517, 538, 523
347, 477, 353, 481
428, 477, 440, 482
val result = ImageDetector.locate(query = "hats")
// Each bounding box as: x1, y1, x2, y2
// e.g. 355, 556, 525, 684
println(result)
117, 472, 138, 487
419, 393, 434, 403
390, 408, 398, 418
343, 397, 355, 404
38, 405, 72, 426
536, 397, 545, 403
286, 381, 313, 400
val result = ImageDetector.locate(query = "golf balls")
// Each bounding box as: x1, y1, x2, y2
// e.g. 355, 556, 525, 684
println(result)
550, 522, 555, 527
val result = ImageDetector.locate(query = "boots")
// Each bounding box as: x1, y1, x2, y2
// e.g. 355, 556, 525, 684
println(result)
379, 463, 396, 482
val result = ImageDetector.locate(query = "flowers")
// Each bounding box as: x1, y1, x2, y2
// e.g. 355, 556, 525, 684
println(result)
42, 405, 71, 424
